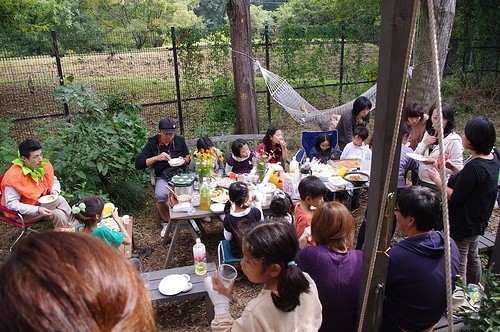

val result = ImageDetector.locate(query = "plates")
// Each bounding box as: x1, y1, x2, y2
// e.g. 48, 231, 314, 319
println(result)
159, 274, 188, 295
328, 176, 348, 186
405, 152, 427, 162
210, 188, 230, 203
37, 195, 59, 204
210, 204, 225, 213
102, 203, 115, 219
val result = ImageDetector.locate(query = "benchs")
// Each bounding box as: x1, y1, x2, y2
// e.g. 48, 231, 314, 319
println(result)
100, 216, 133, 260
151, 133, 267, 229
144, 263, 219, 325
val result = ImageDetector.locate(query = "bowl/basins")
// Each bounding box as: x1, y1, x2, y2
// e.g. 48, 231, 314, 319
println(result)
343, 172, 370, 186
168, 157, 183, 167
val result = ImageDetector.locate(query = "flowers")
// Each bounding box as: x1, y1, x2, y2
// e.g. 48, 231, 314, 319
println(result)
188, 146, 223, 181
299, 163, 312, 176
250, 143, 275, 183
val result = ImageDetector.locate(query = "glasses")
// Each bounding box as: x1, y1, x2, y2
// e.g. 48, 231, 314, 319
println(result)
394, 207, 401, 214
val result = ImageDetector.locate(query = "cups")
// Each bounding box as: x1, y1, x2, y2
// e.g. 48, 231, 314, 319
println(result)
218, 264, 238, 288
177, 194, 192, 203
140, 272, 150, 288
437, 151, 449, 170
123, 215, 129, 224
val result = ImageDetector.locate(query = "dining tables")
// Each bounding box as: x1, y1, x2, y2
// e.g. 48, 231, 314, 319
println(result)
162, 173, 302, 268
322, 166, 372, 212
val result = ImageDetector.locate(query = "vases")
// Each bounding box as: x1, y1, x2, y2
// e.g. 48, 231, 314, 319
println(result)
198, 171, 211, 184
256, 162, 267, 184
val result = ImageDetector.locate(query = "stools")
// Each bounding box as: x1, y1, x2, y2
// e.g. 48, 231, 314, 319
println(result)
217, 239, 242, 281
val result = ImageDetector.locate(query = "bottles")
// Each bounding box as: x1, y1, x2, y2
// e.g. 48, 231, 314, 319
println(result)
359, 142, 372, 177
290, 156, 299, 173
303, 158, 311, 167
193, 238, 208, 276
200, 176, 211, 210
270, 172, 283, 189
257, 151, 266, 181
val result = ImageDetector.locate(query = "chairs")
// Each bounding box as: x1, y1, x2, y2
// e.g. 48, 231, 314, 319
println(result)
0, 175, 47, 251
293, 129, 343, 163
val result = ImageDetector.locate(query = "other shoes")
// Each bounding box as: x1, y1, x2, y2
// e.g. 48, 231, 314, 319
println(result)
202, 217, 211, 223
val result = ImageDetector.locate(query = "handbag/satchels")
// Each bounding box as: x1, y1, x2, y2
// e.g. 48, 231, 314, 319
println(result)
451, 282, 489, 316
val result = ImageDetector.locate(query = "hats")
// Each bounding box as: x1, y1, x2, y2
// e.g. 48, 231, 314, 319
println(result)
159, 118, 177, 134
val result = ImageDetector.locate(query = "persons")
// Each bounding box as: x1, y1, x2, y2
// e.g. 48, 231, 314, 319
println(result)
379, 185, 460, 332
211, 220, 322, 332
72, 195, 143, 275
136, 119, 291, 239
397, 103, 463, 196
427, 118, 500, 332
0, 232, 155, 332
299, 97, 373, 214
0, 139, 79, 229
295, 202, 364, 332
222, 176, 326, 259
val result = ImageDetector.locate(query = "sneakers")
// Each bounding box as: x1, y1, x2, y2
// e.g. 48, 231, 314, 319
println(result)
160, 223, 171, 238
190, 219, 200, 231
